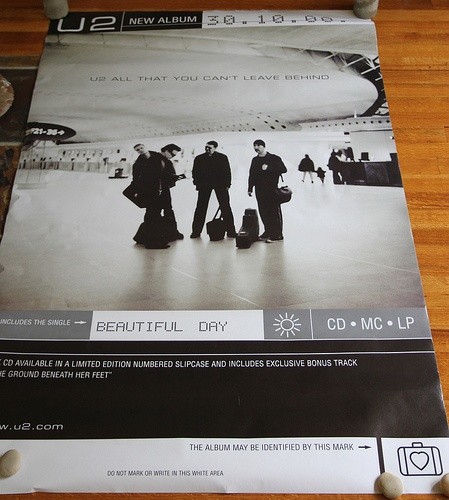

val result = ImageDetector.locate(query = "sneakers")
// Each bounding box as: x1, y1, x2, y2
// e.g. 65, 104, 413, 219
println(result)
265, 233, 283, 243
258, 232, 273, 240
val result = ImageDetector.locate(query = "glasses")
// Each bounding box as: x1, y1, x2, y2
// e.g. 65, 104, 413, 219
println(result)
170, 151, 176, 157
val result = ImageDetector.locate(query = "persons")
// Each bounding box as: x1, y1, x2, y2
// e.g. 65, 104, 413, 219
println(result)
298, 147, 355, 184
128, 144, 185, 242
248, 139, 288, 244
190, 141, 238, 238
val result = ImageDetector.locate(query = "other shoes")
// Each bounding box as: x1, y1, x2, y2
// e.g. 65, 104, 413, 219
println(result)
190, 231, 200, 238
176, 231, 184, 240
227, 230, 238, 237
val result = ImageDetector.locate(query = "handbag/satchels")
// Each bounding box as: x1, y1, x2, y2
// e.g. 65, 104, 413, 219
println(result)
273, 175, 291, 203
133, 217, 174, 249
207, 206, 225, 241
